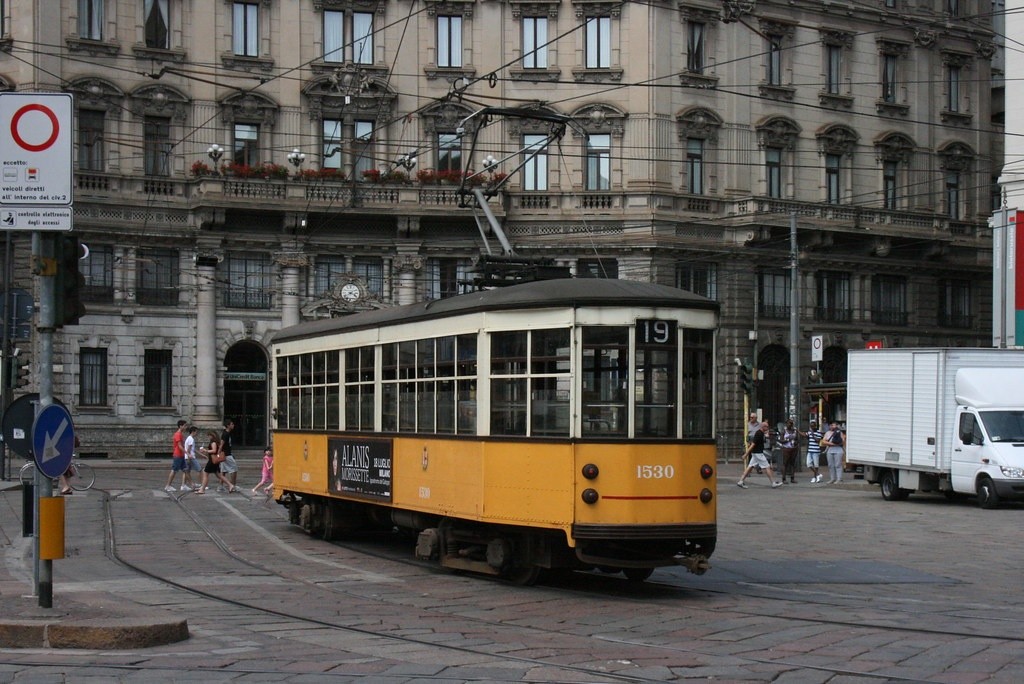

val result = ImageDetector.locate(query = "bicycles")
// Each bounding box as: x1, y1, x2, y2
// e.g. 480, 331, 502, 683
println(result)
19, 456, 95, 491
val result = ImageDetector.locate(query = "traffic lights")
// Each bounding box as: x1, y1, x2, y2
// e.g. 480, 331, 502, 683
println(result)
11, 358, 29, 389
740, 365, 753, 391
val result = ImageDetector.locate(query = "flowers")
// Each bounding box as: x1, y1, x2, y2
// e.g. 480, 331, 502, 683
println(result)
190, 156, 511, 186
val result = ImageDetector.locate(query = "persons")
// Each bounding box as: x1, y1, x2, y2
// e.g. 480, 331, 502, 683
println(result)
748, 413, 762, 474
60, 469, 74, 495
737, 422, 783, 488
252, 446, 273, 495
780, 419, 844, 483
164, 419, 242, 494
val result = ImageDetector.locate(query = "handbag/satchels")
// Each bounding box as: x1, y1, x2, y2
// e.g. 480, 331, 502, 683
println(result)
211, 451, 226, 465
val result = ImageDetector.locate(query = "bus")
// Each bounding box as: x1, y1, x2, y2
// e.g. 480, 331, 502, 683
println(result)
271, 276, 720, 585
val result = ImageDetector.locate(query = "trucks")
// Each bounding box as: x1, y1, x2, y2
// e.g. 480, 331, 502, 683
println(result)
847, 346, 1024, 510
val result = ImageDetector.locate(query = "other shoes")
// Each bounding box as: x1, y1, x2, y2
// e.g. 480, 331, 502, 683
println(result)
736, 482, 749, 489
810, 477, 816, 484
833, 479, 844, 485
791, 480, 797, 483
816, 474, 823, 482
771, 481, 783, 488
782, 481, 788, 484
263, 488, 270, 495
827, 480, 835, 484
165, 482, 240, 494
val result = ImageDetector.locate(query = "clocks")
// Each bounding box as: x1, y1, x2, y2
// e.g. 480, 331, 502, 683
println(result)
327, 271, 370, 306
